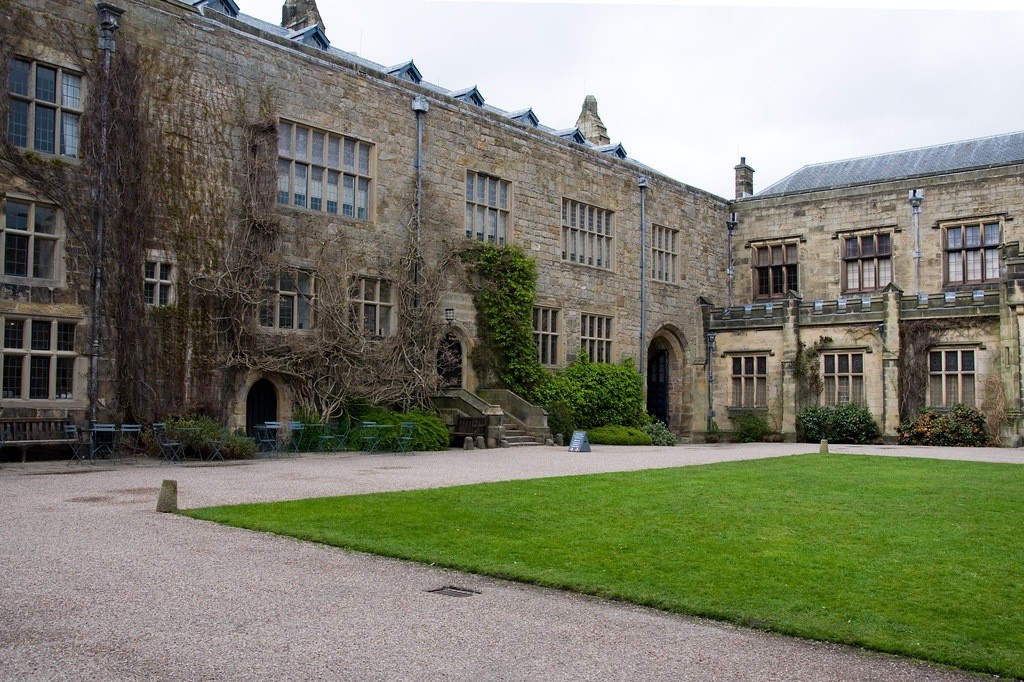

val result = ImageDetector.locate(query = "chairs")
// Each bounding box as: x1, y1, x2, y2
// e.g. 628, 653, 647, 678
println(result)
253, 420, 414, 459
63, 423, 224, 467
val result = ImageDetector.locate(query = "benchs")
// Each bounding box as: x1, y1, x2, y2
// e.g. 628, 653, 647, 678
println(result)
0, 417, 81, 464
